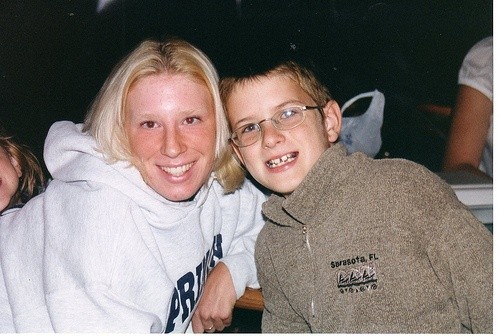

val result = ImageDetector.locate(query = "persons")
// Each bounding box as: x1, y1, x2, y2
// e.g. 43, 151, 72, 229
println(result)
0, 127, 46, 216
218, 59, 493, 334
0, 41, 271, 334
415, 37, 493, 180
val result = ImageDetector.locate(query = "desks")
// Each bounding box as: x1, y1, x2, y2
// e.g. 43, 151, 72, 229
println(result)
432, 164, 493, 224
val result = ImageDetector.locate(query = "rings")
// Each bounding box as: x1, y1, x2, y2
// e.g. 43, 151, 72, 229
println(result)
204, 320, 215, 335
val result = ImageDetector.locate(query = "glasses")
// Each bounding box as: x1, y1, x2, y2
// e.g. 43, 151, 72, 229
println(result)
228, 106, 320, 147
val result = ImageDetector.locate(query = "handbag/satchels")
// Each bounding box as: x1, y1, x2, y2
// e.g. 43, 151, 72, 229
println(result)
336, 89, 385, 159
